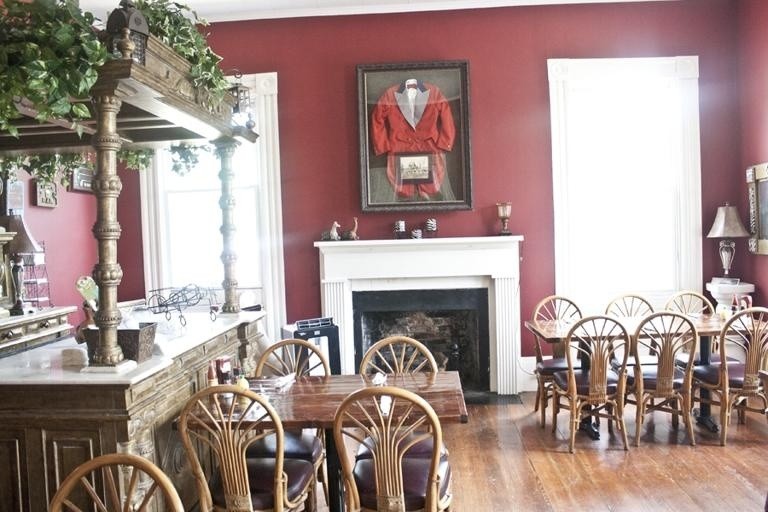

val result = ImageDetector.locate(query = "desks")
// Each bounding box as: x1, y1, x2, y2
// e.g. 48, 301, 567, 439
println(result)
312, 236, 525, 396
706, 282, 755, 360
0, 307, 266, 512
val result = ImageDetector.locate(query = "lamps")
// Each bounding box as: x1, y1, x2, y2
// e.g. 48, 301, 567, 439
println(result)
496, 201, 513, 235
705, 201, 751, 277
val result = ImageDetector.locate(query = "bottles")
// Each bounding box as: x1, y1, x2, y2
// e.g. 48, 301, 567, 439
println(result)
231, 369, 240, 385
222, 375, 233, 408
235, 375, 251, 409
207, 360, 218, 400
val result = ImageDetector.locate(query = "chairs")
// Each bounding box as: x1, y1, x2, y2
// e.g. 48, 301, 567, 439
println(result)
524, 293, 768, 453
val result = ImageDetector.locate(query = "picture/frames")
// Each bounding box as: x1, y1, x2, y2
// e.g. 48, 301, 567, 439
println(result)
745, 162, 768, 255
355, 60, 473, 213
34, 178, 59, 208
68, 164, 99, 194
